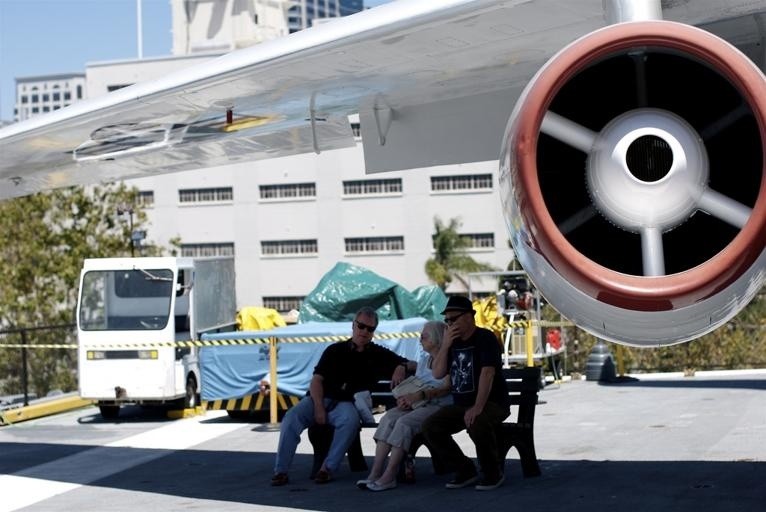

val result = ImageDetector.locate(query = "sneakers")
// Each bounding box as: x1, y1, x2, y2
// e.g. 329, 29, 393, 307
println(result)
314, 469, 334, 484
444, 472, 479, 490
269, 472, 289, 487
475, 474, 505, 491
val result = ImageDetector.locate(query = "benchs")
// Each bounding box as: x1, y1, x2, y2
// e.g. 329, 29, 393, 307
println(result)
305, 363, 542, 480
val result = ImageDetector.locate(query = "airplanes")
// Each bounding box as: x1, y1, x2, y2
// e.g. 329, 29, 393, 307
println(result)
0, 1, 764, 356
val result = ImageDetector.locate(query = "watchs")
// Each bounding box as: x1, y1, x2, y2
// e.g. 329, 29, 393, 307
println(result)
399, 363, 408, 374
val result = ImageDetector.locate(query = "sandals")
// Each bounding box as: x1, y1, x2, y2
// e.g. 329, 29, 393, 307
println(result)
366, 480, 398, 492
355, 479, 376, 489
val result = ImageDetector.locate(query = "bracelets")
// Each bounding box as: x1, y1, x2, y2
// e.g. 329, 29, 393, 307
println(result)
420, 390, 425, 400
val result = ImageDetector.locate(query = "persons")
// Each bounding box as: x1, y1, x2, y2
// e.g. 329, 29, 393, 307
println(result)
522, 287, 534, 311
272, 308, 418, 486
420, 295, 510, 490
356, 321, 452, 492
546, 328, 562, 383
505, 282, 520, 310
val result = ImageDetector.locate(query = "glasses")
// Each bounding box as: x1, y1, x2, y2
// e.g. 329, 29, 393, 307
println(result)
355, 319, 377, 333
444, 312, 468, 324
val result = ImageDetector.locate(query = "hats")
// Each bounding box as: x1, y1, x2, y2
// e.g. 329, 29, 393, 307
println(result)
440, 294, 476, 315
